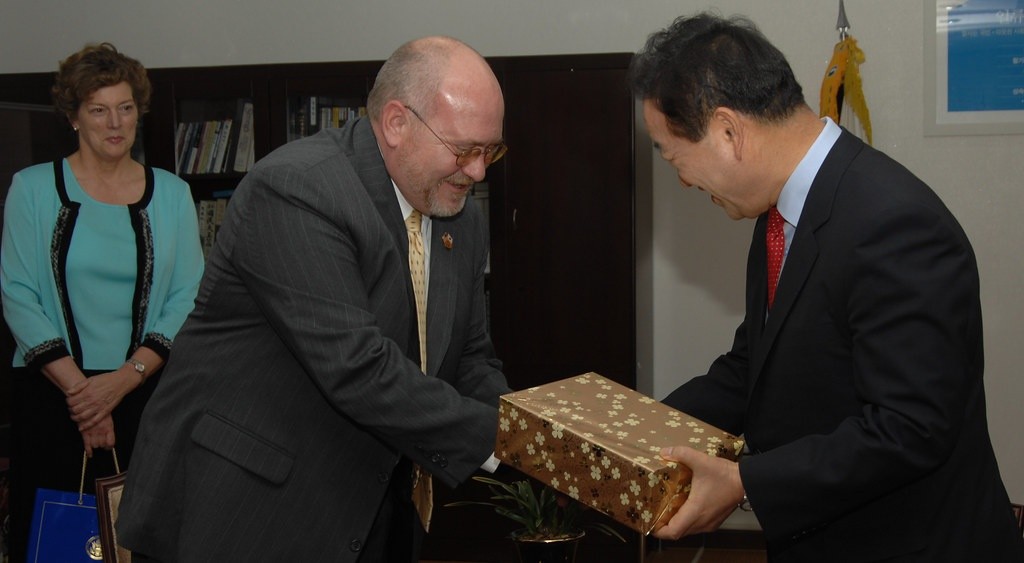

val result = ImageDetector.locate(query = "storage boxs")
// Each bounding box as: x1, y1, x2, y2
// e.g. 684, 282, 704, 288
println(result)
495, 373, 745, 536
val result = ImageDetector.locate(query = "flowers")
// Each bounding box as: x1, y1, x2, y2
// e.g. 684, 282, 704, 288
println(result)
444, 477, 626, 546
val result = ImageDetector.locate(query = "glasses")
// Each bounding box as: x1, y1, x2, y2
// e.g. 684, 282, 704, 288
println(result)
401, 103, 509, 167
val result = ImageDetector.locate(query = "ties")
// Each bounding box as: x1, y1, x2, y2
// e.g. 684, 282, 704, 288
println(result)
403, 207, 434, 533
766, 208, 785, 314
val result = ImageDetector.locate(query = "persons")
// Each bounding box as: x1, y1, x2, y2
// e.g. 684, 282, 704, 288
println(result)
111, 36, 513, 563
622, 11, 1024, 563
0, 39, 205, 563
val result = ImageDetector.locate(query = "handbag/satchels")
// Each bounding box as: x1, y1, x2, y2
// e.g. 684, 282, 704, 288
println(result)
28, 444, 120, 563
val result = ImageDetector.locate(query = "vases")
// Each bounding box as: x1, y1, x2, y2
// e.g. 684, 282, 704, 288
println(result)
508, 527, 587, 563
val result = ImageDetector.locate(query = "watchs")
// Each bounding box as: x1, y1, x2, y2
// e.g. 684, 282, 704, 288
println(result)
127, 359, 147, 382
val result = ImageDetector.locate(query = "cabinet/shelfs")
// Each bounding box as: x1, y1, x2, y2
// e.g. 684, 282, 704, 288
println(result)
0, 52, 656, 401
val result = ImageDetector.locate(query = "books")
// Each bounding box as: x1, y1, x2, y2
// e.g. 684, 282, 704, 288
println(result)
288, 93, 367, 141
196, 190, 236, 264
175, 100, 255, 175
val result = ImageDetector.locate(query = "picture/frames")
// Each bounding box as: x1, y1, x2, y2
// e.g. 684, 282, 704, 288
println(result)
924, 0, 1024, 137
